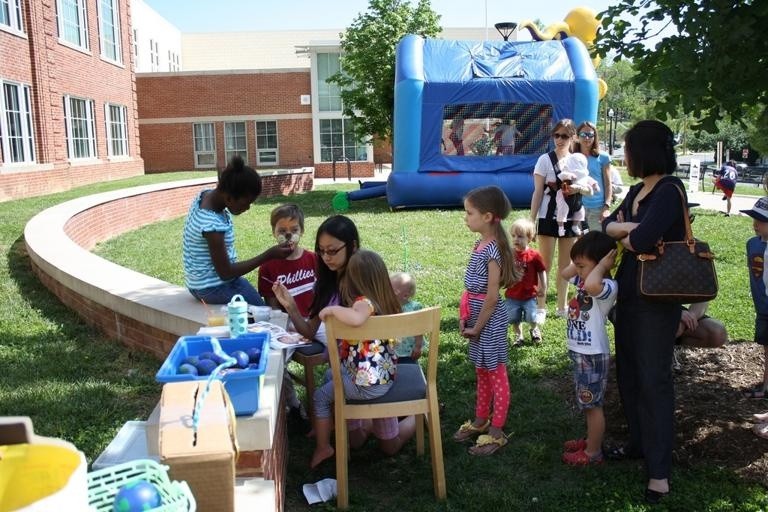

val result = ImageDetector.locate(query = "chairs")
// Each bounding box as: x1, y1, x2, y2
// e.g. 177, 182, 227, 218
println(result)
291, 340, 331, 421
325, 306, 448, 510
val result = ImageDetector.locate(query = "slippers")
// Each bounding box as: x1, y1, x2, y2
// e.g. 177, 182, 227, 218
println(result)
453, 419, 491, 441
468, 432, 514, 456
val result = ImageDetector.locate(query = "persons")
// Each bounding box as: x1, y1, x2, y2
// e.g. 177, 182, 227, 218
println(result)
483, 117, 524, 155
740, 198, 768, 399
752, 168, 768, 440
310, 250, 403, 470
604, 119, 692, 511
608, 203, 727, 348
258, 204, 319, 317
561, 230, 618, 466
712, 159, 737, 217
529, 118, 594, 326
183, 156, 295, 306
453, 185, 521, 455
556, 155, 600, 235
509, 219, 547, 346
576, 121, 612, 234
451, 118, 465, 156
390, 272, 425, 359
272, 215, 360, 338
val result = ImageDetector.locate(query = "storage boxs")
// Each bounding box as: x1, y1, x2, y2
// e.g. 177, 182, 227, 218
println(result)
155, 332, 269, 416
146, 380, 241, 512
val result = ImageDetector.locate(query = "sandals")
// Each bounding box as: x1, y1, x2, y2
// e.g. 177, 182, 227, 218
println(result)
565, 436, 586, 452
562, 448, 605, 465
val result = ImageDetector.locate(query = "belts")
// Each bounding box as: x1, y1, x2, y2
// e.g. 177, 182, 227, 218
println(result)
460, 290, 488, 321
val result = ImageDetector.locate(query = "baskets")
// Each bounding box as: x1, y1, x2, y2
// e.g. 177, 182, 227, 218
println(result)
84, 458, 197, 511
0, 416, 88, 512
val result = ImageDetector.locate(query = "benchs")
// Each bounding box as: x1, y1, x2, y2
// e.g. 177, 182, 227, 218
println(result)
674, 164, 707, 192
712, 166, 768, 194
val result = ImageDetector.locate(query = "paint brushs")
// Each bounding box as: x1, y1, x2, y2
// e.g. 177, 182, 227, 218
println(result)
260, 276, 278, 285
192, 288, 214, 319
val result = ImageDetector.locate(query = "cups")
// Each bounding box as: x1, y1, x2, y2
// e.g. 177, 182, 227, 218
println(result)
252, 307, 272, 323
207, 310, 224, 327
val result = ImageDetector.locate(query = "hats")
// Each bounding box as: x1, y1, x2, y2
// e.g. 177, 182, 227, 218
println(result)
687, 202, 700, 208
739, 197, 768, 222
559, 153, 589, 176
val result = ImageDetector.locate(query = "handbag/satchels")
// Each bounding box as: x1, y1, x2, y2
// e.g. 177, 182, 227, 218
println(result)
637, 183, 719, 303
609, 165, 622, 202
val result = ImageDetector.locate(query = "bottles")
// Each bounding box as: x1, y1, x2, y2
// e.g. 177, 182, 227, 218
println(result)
227, 294, 249, 339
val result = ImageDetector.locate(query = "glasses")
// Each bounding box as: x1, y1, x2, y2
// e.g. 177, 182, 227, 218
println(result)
317, 243, 346, 257
579, 131, 594, 138
689, 214, 695, 224
553, 133, 569, 139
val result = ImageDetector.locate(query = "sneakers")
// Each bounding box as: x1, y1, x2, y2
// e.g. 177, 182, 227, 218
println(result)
723, 196, 727, 200
745, 389, 767, 399
536, 304, 546, 325
754, 423, 768, 439
530, 328, 542, 344
292, 404, 311, 432
725, 214, 729, 217
512, 334, 524, 347
556, 307, 569, 317
753, 410, 768, 423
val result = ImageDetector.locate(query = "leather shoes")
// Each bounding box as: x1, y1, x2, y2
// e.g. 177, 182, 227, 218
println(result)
645, 476, 670, 503
614, 444, 626, 459
